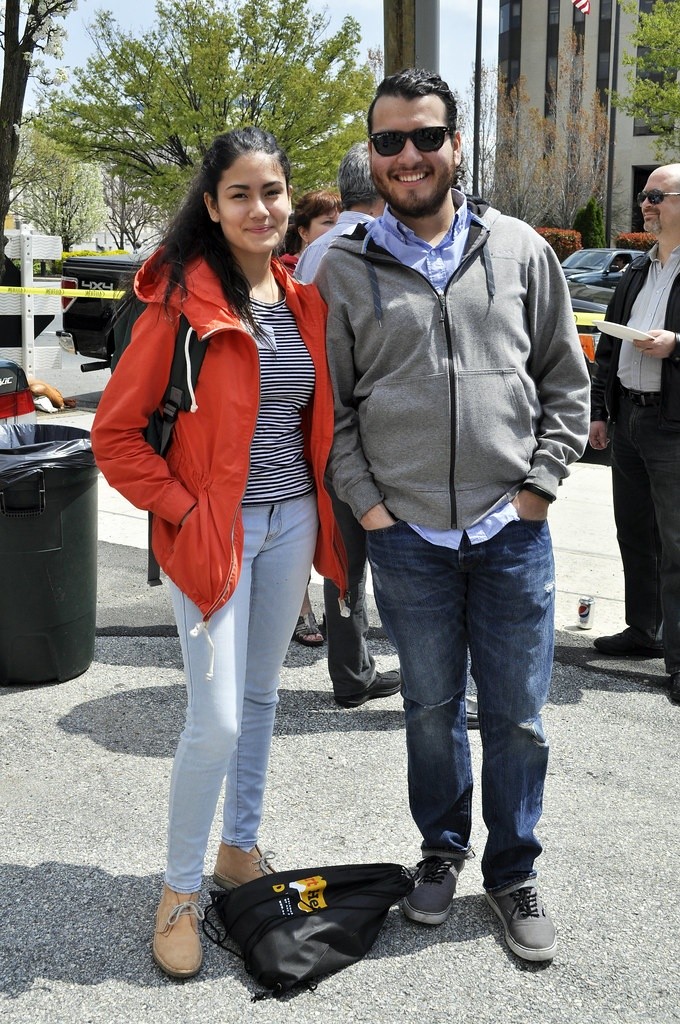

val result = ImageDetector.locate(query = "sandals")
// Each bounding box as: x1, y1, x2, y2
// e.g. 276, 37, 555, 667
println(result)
293, 613, 325, 647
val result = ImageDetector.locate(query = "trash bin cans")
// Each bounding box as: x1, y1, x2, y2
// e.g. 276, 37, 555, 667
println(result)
0, 424, 99, 686
0, 356, 37, 424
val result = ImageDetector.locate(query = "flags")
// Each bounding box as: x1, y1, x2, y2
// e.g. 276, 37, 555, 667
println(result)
571, 0, 590, 15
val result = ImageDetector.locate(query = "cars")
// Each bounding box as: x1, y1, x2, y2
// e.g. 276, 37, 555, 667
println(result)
561, 248, 647, 289
566, 282, 615, 377
0, 356, 37, 425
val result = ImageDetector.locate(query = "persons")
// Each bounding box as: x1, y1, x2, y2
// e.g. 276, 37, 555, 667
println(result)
291, 140, 481, 730
313, 65, 591, 958
274, 188, 326, 647
588, 162, 680, 703
91, 122, 350, 978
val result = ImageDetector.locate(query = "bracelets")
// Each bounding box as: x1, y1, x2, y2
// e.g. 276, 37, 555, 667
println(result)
522, 483, 555, 503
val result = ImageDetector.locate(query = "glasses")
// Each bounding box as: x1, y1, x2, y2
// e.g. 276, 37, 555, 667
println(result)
368, 126, 451, 156
636, 189, 680, 207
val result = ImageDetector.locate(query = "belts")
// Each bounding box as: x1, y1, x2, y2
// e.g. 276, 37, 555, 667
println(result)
621, 386, 660, 407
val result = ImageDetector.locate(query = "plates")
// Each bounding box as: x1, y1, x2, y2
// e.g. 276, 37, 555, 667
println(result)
592, 320, 655, 342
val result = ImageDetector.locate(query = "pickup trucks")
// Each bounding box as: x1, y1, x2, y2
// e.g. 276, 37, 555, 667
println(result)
56, 256, 145, 372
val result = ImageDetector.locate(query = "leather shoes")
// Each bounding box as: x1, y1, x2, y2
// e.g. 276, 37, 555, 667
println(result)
464, 698, 479, 727
335, 668, 401, 706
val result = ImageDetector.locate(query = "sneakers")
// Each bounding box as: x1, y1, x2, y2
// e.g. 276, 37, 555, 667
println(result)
152, 884, 206, 977
485, 886, 557, 961
403, 855, 467, 924
212, 842, 278, 890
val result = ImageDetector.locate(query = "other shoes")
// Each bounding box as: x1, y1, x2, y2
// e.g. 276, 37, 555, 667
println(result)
594, 633, 665, 658
670, 672, 680, 698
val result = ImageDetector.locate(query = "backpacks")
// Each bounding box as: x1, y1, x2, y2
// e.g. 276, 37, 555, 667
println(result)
202, 863, 415, 1002
80, 286, 210, 456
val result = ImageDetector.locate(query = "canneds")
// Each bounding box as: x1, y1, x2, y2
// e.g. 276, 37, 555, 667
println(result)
577, 596, 595, 629
